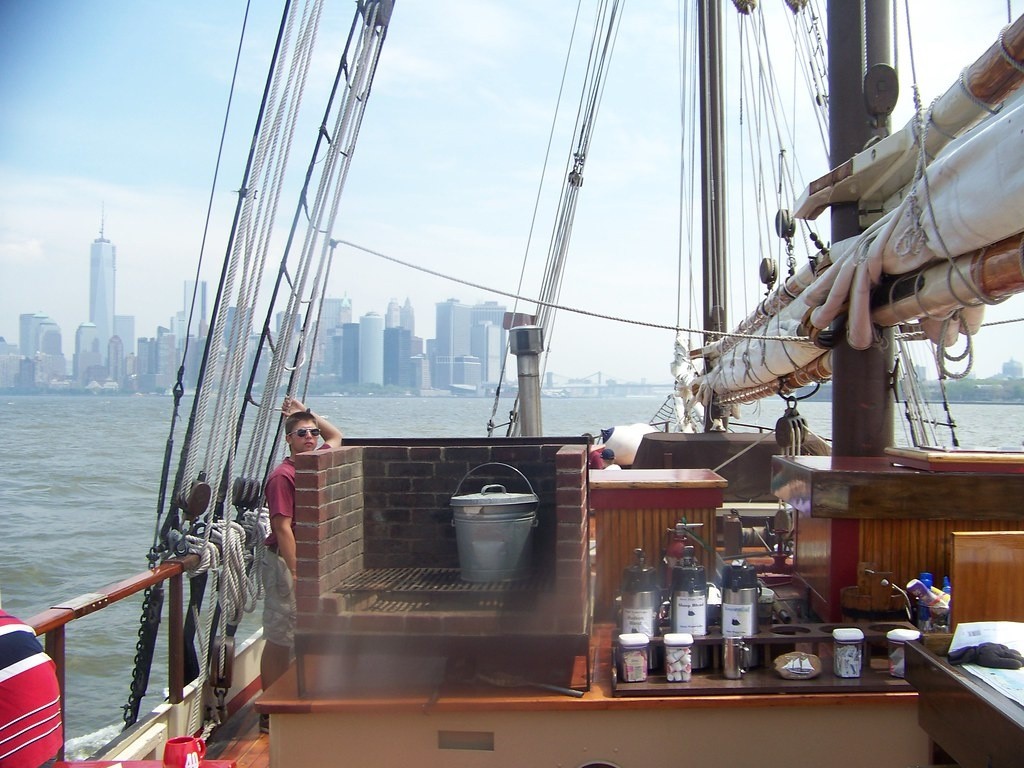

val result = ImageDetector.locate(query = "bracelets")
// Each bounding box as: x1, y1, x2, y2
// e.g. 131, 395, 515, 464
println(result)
306, 408, 310, 414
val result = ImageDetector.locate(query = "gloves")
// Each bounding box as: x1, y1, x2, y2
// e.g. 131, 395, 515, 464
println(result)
948, 641, 1024, 669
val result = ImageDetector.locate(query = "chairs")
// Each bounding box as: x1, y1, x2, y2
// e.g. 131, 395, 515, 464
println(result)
904, 531, 1024, 768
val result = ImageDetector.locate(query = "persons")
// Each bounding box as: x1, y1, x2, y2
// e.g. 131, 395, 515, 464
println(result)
260, 395, 343, 733
581, 433, 622, 470
0, 607, 65, 768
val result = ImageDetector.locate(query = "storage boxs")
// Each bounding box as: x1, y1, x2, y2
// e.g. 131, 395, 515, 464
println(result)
887, 628, 922, 678
618, 633, 650, 682
832, 627, 864, 679
663, 633, 695, 681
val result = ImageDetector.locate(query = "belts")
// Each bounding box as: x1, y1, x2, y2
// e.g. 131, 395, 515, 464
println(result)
267, 543, 283, 557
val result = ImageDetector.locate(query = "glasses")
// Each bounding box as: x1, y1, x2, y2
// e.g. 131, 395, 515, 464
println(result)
288, 428, 322, 438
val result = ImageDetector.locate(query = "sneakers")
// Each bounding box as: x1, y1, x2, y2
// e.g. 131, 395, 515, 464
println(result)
259, 713, 270, 734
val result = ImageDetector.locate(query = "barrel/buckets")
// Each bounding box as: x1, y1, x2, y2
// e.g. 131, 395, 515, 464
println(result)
449, 462, 540, 582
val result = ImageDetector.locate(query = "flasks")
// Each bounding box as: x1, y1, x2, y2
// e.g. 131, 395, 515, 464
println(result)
722, 559, 761, 667
622, 549, 658, 669
671, 546, 709, 669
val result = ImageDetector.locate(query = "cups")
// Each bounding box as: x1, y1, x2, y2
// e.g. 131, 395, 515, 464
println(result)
757, 588, 774, 625
163, 736, 206, 768
916, 603, 950, 633
722, 635, 750, 679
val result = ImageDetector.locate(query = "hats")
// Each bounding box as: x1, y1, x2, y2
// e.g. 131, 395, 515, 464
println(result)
600, 448, 616, 460
